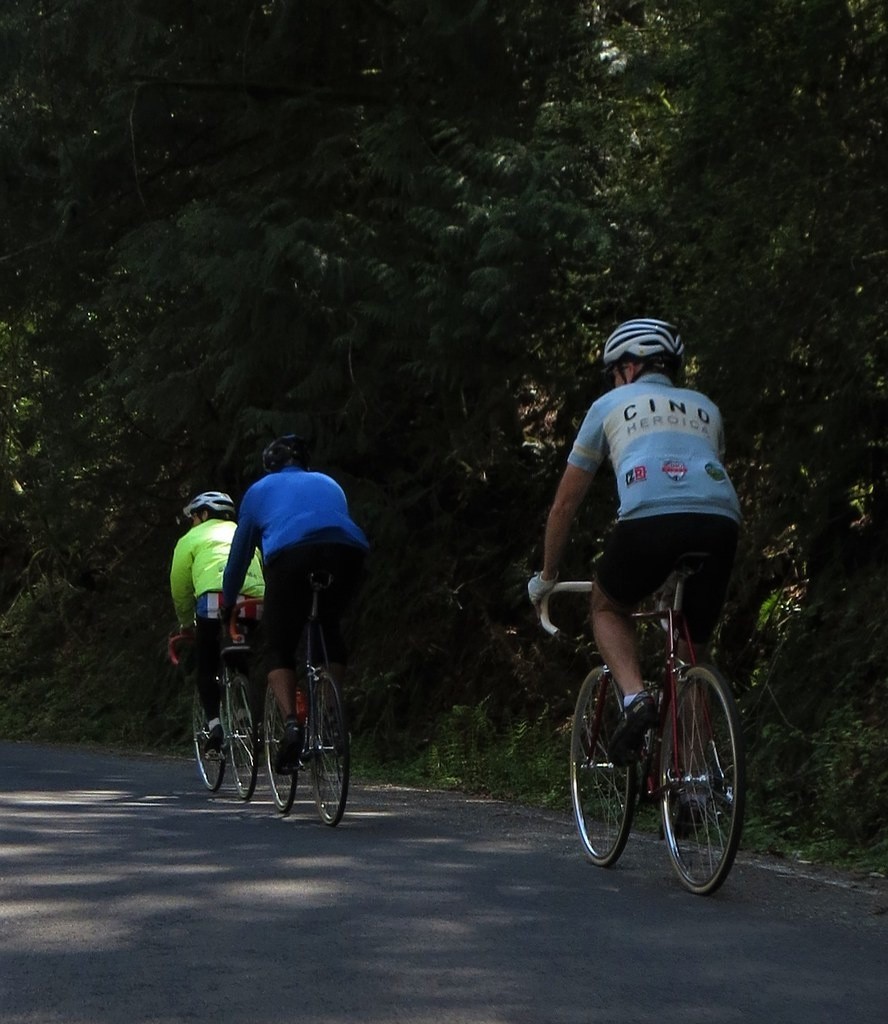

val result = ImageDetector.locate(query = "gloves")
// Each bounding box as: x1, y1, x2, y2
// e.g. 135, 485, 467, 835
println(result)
179, 623, 195, 637
218, 605, 241, 623
527, 571, 559, 620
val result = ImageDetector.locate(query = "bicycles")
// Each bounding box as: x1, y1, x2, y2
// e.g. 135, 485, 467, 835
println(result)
167, 631, 259, 801
540, 580, 747, 899
230, 596, 350, 828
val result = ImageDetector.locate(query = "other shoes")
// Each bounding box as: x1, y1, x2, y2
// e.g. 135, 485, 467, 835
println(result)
238, 718, 252, 741
326, 705, 352, 749
274, 723, 304, 776
204, 724, 224, 754
675, 795, 704, 838
607, 691, 657, 768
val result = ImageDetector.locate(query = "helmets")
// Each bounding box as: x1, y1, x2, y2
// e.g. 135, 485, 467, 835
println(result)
601, 318, 685, 366
183, 491, 236, 518
263, 435, 314, 473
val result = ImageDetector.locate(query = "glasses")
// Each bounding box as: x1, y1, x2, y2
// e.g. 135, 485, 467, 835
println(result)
191, 514, 198, 520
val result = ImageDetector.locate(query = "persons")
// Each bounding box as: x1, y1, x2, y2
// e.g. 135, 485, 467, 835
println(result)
169, 491, 265, 753
222, 434, 371, 771
527, 317, 742, 835
